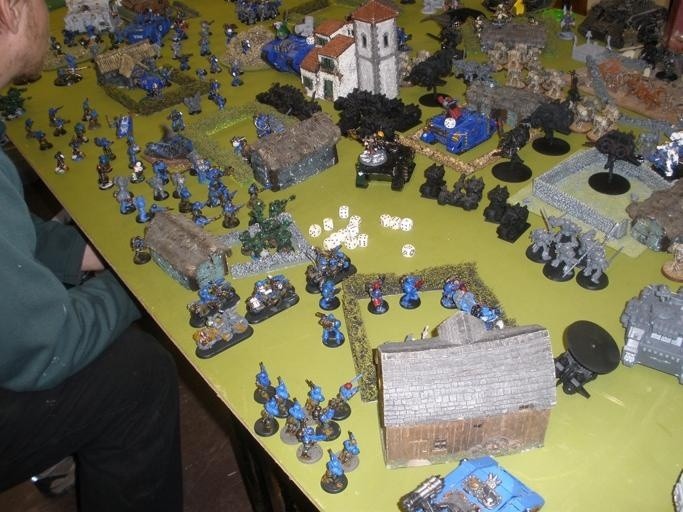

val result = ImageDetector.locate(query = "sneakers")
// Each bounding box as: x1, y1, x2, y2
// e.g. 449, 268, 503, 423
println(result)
34, 457, 76, 498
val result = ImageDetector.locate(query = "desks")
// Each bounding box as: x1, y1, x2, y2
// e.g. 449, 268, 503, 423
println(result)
2, 0, 682, 512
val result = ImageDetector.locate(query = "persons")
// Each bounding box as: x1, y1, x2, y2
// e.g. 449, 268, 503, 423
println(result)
0, 1, 184, 512
1, 0, 682, 507
